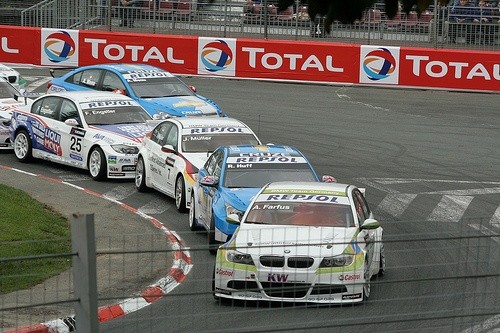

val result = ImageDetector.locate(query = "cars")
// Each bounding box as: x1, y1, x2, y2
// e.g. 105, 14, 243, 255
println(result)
46, 64, 226, 119
135, 117, 263, 212
212, 181, 387, 308
9, 90, 159, 182
189, 144, 337, 255
0, 63, 34, 151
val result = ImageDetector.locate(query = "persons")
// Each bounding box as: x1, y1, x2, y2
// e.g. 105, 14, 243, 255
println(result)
118, 0, 139, 28
450, 0, 500, 44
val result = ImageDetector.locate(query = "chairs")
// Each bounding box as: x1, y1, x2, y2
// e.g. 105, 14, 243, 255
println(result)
111, 0, 433, 34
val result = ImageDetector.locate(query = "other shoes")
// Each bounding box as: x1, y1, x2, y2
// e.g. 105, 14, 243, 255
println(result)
490, 43, 494, 48
120, 21, 127, 27
466, 41, 469, 46
448, 39, 456, 43
129, 21, 135, 29
472, 42, 475, 46
